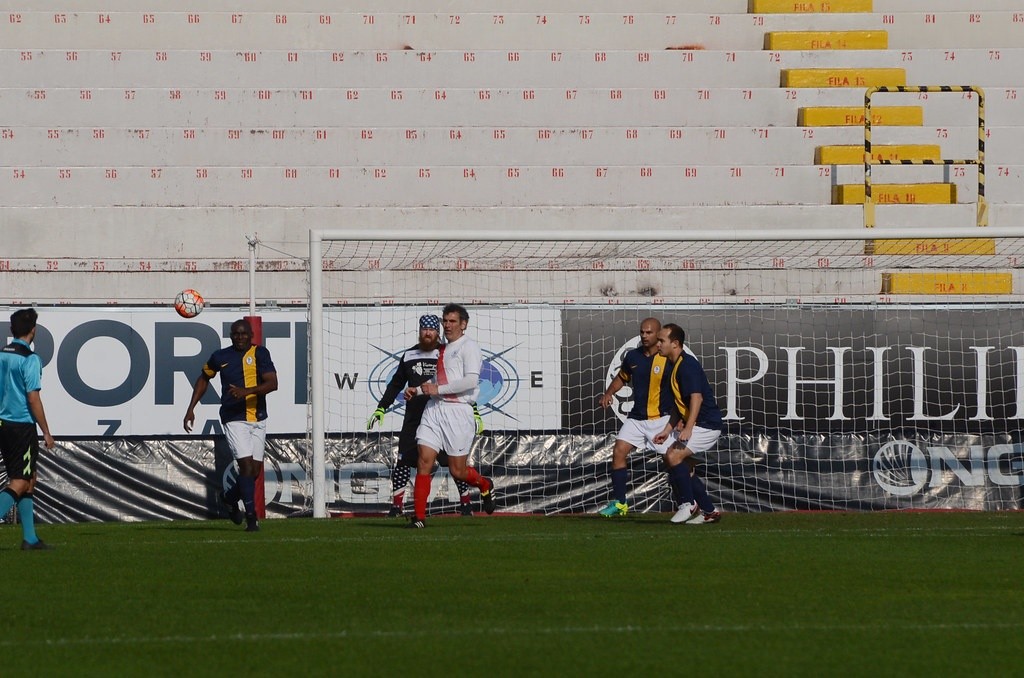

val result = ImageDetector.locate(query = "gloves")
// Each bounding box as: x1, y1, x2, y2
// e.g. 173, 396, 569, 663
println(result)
470, 402, 483, 435
366, 408, 385, 431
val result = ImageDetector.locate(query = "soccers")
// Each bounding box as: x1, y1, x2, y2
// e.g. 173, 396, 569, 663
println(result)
174, 289, 203, 318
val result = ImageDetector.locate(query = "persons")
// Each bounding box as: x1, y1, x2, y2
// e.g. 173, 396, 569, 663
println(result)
403, 304, 496, 528
597, 317, 684, 517
367, 314, 482, 517
653, 323, 722, 525
0, 308, 55, 550
183, 320, 278, 530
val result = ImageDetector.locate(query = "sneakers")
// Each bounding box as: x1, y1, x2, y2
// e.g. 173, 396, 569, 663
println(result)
405, 518, 426, 529
244, 510, 260, 532
670, 500, 722, 525
479, 477, 495, 515
21, 538, 56, 551
460, 503, 474, 517
218, 490, 243, 526
387, 508, 405, 518
597, 499, 628, 519
